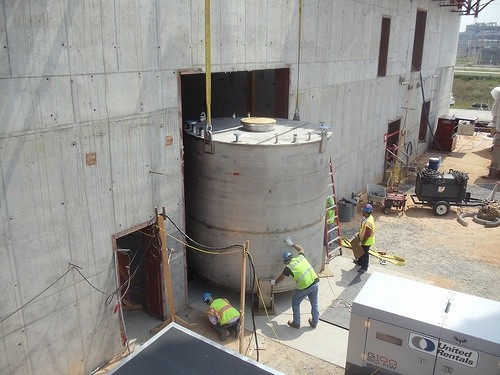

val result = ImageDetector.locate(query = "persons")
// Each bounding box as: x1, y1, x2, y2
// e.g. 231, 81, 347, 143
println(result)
202, 292, 240, 342
270, 236, 320, 328
352, 204, 375, 274
323, 197, 335, 246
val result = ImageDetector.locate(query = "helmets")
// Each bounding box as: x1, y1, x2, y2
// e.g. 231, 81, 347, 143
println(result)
282, 251, 292, 261
361, 204, 373, 213
202, 292, 212, 302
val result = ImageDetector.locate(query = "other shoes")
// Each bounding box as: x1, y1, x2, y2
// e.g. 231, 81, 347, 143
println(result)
354, 260, 362, 266
288, 320, 300, 328
309, 318, 316, 328
357, 268, 367, 272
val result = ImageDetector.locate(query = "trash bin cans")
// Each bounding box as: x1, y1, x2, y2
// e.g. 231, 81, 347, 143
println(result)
338, 197, 357, 222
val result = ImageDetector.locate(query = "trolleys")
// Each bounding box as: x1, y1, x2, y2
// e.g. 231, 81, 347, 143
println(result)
411, 191, 486, 217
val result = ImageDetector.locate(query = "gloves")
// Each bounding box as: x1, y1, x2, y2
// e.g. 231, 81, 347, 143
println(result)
271, 280, 276, 286
283, 235, 294, 247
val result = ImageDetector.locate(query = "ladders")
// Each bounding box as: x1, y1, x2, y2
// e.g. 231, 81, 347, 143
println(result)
326, 155, 342, 257
